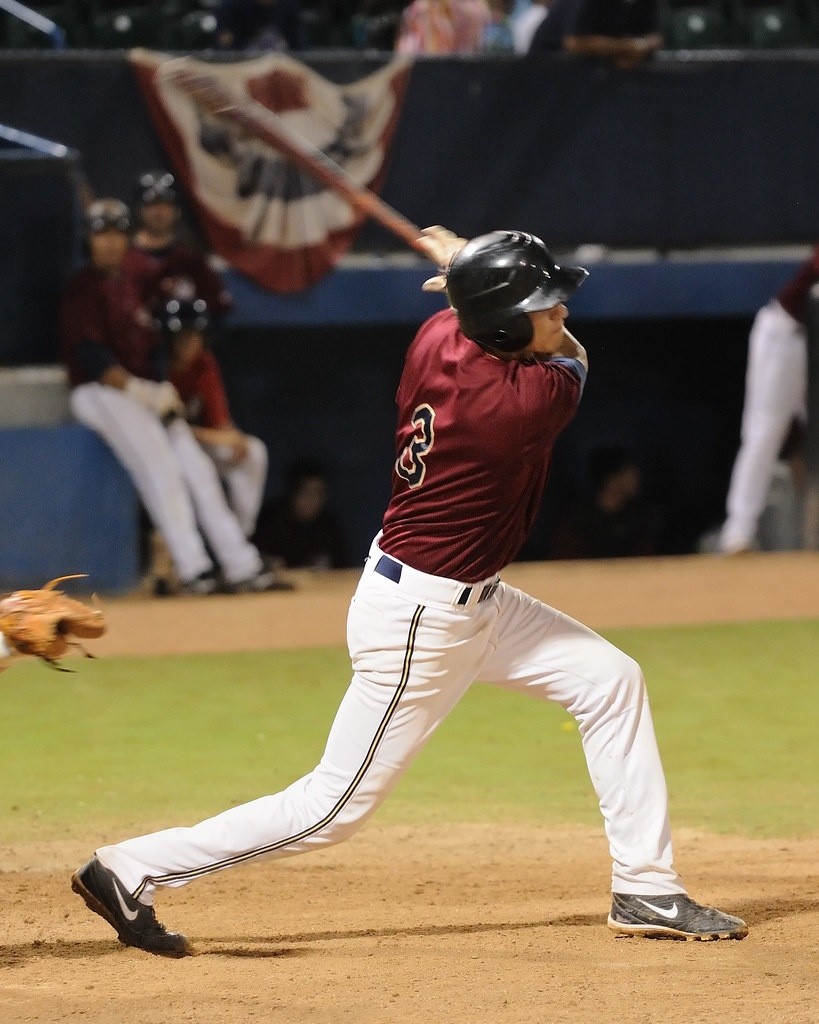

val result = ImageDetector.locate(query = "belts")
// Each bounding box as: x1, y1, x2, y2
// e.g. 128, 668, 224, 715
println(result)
374, 556, 501, 604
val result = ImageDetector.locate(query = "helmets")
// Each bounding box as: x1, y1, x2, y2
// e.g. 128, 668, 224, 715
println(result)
138, 172, 182, 208
446, 231, 588, 351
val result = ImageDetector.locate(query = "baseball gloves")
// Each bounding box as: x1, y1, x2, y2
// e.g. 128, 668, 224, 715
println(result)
0, 571, 106, 677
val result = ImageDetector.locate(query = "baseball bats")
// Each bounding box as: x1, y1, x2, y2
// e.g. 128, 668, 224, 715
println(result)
159, 55, 419, 244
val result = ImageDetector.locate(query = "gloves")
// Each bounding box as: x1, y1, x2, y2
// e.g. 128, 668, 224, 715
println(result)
415, 220, 468, 292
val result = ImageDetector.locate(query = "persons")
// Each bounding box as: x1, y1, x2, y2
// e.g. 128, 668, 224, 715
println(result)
62, 0, 819, 600
70, 226, 749, 957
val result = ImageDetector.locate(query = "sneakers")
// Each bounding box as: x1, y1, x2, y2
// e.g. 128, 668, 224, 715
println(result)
607, 891, 748, 941
71, 856, 186, 952
